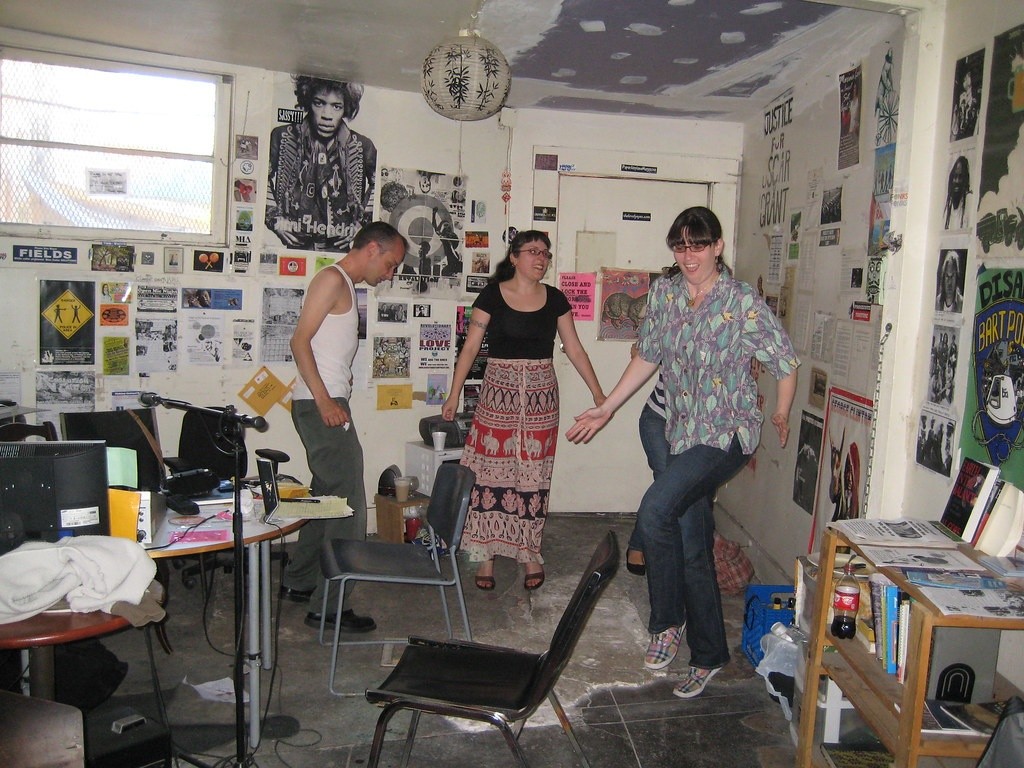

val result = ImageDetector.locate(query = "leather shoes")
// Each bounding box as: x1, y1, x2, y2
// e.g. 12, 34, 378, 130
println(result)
305, 609, 376, 633
278, 584, 316, 602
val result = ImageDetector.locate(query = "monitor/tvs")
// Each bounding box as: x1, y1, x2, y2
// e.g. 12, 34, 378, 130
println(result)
0, 440, 111, 556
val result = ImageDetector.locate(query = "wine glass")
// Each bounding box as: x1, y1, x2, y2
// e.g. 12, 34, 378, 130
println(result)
405, 475, 419, 498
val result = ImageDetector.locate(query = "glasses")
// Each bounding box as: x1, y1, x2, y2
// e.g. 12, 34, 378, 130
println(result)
513, 247, 552, 259
668, 240, 715, 253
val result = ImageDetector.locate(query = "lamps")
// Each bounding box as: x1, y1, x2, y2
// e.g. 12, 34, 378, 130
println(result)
421, 28, 511, 123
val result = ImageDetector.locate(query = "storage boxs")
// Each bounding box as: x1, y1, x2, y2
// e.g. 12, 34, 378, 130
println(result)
374, 491, 431, 542
741, 583, 796, 668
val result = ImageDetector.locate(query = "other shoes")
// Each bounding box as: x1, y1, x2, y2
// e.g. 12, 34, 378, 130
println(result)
673, 665, 722, 698
644, 620, 687, 669
626, 549, 645, 576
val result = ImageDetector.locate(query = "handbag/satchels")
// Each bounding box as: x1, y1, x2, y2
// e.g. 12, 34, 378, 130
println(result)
713, 531, 753, 593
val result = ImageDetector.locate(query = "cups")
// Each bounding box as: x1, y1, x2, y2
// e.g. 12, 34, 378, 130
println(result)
393, 477, 410, 502
432, 431, 447, 451
771, 623, 796, 645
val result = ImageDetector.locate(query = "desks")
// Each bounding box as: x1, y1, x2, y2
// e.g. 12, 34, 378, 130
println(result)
22, 488, 312, 748
0, 579, 176, 768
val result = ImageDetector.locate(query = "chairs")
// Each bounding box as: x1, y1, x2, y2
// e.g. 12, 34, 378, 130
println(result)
0, 408, 165, 492
320, 461, 475, 696
364, 530, 621, 768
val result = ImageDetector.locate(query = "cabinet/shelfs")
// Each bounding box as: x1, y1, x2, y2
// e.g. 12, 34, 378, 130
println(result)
794, 524, 1024, 768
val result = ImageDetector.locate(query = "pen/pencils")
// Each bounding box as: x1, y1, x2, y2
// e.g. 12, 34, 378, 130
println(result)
281, 498, 320, 503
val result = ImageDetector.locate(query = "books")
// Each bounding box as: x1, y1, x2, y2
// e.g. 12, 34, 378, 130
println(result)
869, 580, 1001, 703
940, 456, 1024, 558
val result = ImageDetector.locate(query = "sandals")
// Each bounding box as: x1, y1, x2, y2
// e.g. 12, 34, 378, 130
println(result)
524, 562, 543, 589
474, 566, 495, 589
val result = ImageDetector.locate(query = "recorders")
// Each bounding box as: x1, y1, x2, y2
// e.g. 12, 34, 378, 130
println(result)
420, 413, 474, 448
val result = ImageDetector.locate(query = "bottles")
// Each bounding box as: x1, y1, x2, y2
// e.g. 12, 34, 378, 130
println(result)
788, 598, 795, 610
830, 563, 860, 640
774, 597, 781, 610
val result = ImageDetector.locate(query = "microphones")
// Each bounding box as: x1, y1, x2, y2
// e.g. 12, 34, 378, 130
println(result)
138, 391, 157, 407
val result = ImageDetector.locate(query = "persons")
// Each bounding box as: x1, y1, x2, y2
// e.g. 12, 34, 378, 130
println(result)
929, 333, 958, 405
264, 75, 377, 253
944, 156, 971, 228
394, 207, 463, 282
829, 426, 860, 522
935, 251, 963, 313
625, 343, 667, 576
442, 231, 604, 588
280, 221, 409, 632
188, 289, 211, 309
417, 170, 445, 194
917, 415, 955, 478
567, 206, 803, 698
